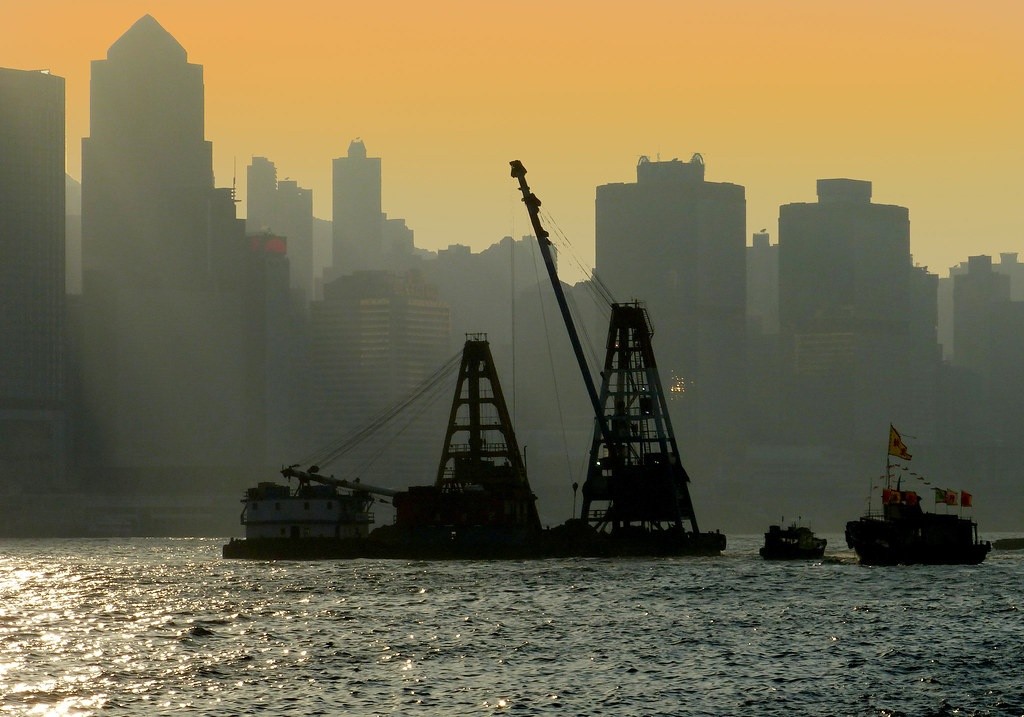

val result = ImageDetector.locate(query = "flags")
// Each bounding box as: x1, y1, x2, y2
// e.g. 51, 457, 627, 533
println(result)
935, 487, 946, 503
946, 488, 958, 505
961, 490, 973, 507
882, 489, 917, 506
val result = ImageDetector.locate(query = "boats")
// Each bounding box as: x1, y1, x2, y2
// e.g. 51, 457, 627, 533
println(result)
760, 515, 828, 561
222, 158, 729, 557
845, 423, 991, 564
